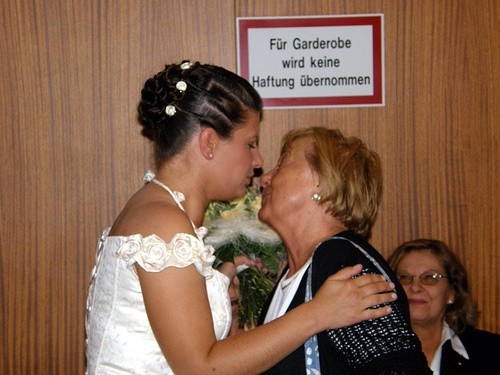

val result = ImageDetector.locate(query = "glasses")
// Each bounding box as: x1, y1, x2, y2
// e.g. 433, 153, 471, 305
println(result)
394, 270, 449, 286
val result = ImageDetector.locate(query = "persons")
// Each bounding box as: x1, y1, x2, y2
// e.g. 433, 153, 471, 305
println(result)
387, 238, 500, 375
253, 124, 434, 374
82, 59, 397, 375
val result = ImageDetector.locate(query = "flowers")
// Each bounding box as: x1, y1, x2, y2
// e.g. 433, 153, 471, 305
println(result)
202, 184, 289, 331
